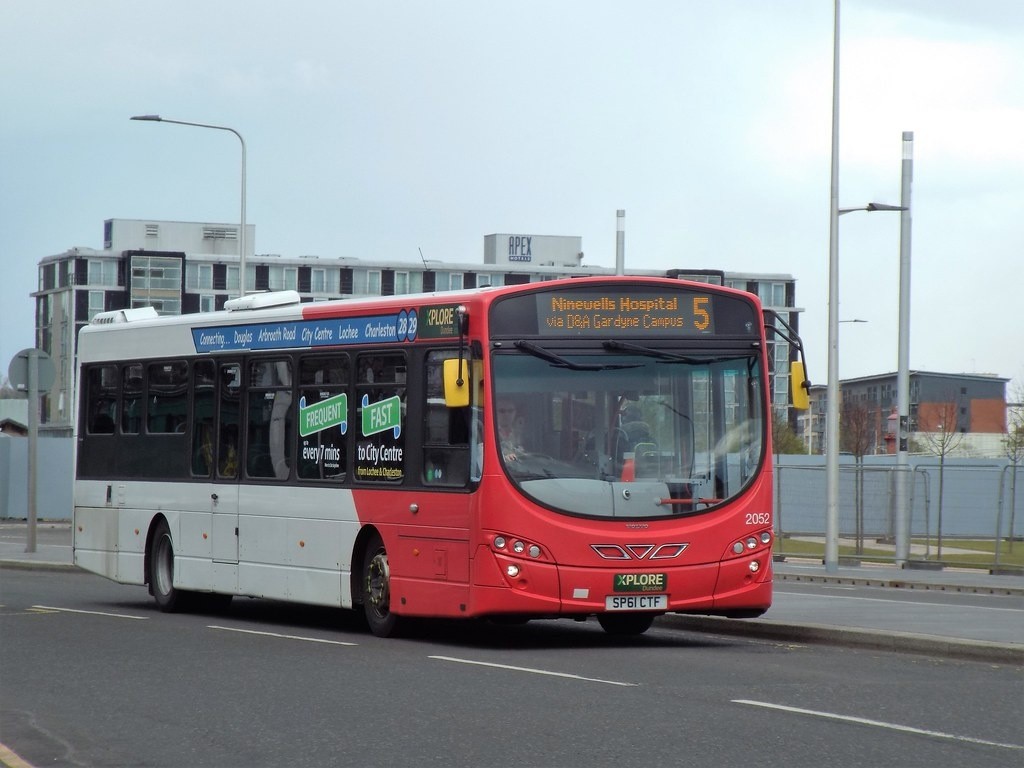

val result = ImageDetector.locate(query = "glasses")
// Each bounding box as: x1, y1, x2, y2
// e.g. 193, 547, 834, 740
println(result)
497, 408, 515, 414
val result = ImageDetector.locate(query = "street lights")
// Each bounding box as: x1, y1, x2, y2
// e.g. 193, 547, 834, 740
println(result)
132, 114, 245, 294
826, 202, 908, 570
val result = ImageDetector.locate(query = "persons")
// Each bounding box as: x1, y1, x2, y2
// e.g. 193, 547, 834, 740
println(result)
497, 396, 529, 462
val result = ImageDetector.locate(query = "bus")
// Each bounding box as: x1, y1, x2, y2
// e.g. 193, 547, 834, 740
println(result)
72, 273, 814, 637
